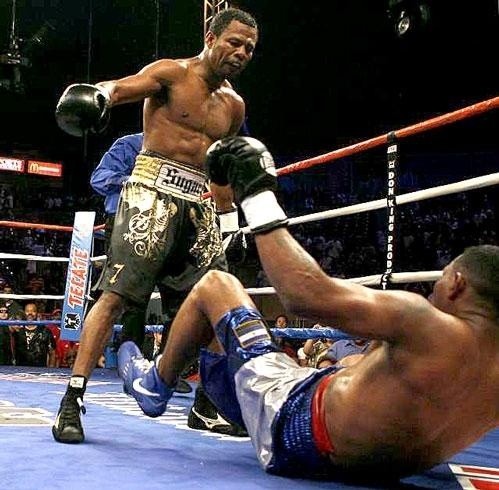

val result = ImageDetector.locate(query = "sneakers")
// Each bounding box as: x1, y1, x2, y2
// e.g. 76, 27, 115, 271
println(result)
176, 379, 192, 394
188, 387, 249, 437
52, 375, 85, 444
116, 341, 167, 418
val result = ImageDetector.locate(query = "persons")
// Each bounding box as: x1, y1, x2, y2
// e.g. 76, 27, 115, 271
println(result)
0, 209, 80, 369
52, 7, 258, 444
295, 191, 499, 296
116, 138, 499, 487
273, 314, 384, 371
88, 131, 169, 361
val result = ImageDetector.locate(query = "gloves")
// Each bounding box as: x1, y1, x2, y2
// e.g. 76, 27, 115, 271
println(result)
204, 135, 289, 234
55, 83, 111, 139
